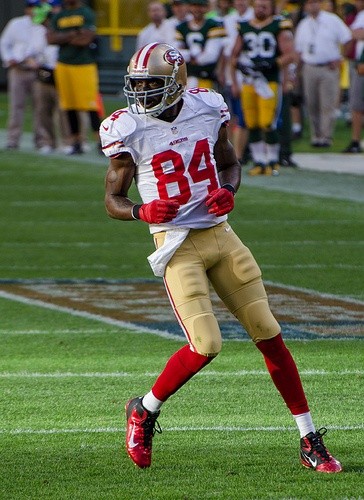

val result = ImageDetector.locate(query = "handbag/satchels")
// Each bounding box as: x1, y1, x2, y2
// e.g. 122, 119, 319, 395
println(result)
36, 67, 54, 85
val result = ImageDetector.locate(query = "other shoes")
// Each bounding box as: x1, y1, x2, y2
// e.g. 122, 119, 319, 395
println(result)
39, 146, 52, 154
247, 161, 264, 175
312, 143, 328, 147
62, 145, 72, 154
341, 140, 360, 152
265, 162, 280, 175
73, 144, 82, 153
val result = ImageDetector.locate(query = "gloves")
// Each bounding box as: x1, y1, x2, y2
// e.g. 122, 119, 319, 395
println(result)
205, 188, 234, 217
139, 199, 179, 224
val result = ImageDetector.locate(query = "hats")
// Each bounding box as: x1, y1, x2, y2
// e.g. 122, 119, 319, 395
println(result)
48, 0, 61, 7
25, 0, 40, 7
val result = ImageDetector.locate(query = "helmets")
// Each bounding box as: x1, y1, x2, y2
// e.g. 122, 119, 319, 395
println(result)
123, 42, 187, 117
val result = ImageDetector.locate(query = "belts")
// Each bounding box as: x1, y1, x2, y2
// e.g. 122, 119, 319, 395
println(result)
305, 63, 333, 67
15, 66, 41, 72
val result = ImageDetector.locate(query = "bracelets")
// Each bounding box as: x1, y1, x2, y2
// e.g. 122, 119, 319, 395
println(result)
221, 184, 236, 196
131, 204, 143, 221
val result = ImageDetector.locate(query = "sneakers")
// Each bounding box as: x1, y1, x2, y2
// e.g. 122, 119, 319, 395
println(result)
299, 427, 342, 473
126, 396, 162, 468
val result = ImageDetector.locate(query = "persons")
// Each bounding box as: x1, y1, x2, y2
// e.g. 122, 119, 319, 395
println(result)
99, 41, 345, 474
0, 0, 364, 175
